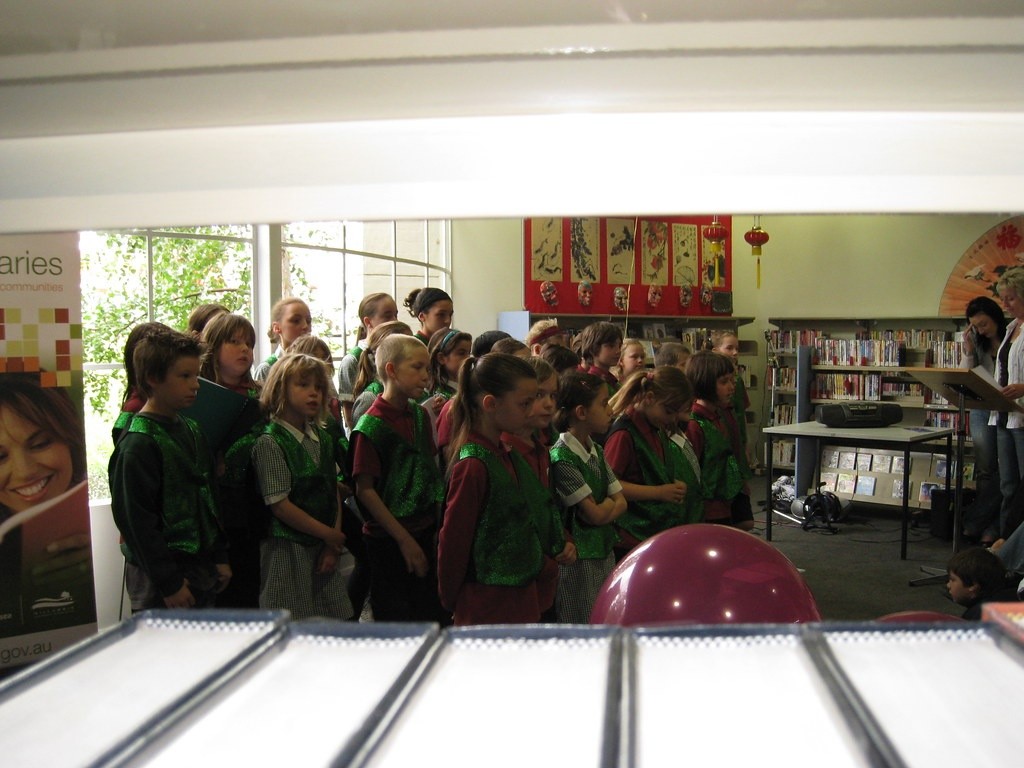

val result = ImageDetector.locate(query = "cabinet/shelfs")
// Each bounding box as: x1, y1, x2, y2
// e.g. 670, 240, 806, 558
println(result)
764, 316, 977, 515
529, 312, 760, 427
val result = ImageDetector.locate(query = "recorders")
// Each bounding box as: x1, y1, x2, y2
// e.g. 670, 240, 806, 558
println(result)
791, 497, 838, 520
814, 401, 904, 429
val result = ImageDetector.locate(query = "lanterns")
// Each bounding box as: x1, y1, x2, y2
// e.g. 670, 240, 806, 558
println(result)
703, 222, 729, 286
744, 226, 769, 289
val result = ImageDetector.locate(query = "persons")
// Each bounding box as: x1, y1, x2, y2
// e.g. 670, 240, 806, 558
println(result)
107, 287, 751, 629
987, 267, 1024, 555
946, 547, 1018, 622
957, 297, 1010, 547
0, 372, 92, 584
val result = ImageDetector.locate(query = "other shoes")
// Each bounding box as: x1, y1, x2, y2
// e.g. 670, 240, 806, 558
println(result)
980, 534, 994, 547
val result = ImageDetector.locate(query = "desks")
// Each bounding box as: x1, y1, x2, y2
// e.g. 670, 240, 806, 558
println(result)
763, 420, 954, 560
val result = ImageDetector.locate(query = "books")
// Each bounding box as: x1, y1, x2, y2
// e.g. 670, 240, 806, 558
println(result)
0, 479, 92, 602
763, 328, 974, 501
